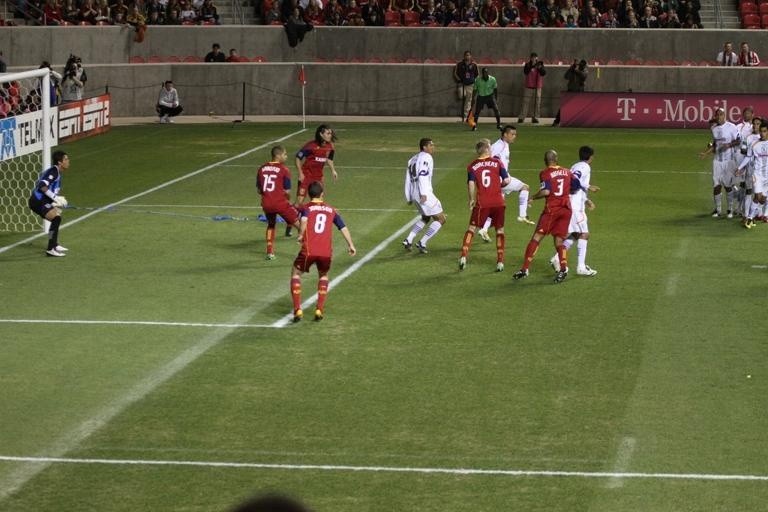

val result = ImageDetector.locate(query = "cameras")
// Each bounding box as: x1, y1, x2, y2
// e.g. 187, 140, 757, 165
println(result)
536, 61, 545, 67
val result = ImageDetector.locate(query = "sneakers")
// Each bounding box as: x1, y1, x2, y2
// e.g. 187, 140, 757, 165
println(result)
266, 231, 323, 322
518, 119, 523, 123
402, 216, 598, 284
167, 118, 174, 123
46, 248, 66, 257
497, 125, 503, 129
532, 120, 539, 123
472, 126, 477, 131
56, 245, 69, 252
711, 208, 768, 229
160, 117, 166, 124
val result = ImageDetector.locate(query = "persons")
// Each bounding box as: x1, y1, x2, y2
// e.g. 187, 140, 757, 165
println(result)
512, 149, 579, 286
478, 124, 537, 245
283, 125, 340, 243
454, 137, 511, 271
714, 41, 737, 67
551, 58, 589, 128
400, 137, 446, 253
472, 67, 504, 131
204, 42, 230, 63
290, 179, 355, 324
224, 48, 240, 63
257, 2, 702, 29
0, 51, 87, 125
451, 50, 479, 124
153, 77, 184, 125
550, 145, 602, 277
735, 42, 761, 67
518, 52, 547, 125
255, 144, 300, 262
27, 149, 72, 262
25, 1, 220, 26
697, 98, 768, 230
